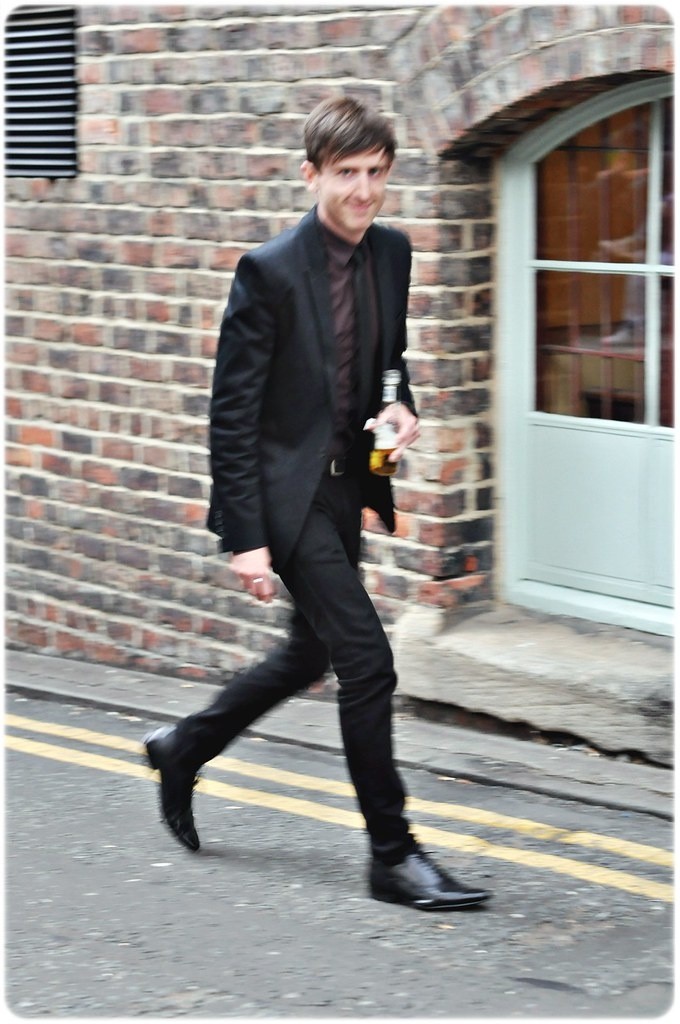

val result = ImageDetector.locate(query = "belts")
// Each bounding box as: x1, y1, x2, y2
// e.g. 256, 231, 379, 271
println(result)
323, 455, 346, 476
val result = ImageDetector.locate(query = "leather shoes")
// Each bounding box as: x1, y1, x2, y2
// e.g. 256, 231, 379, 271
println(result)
145, 725, 201, 851
370, 848, 490, 910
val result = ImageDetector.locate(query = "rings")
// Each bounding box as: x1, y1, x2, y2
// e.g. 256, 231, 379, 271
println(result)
253, 577, 263, 584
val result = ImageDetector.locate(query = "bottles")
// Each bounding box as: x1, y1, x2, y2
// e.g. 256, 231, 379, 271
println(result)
369, 370, 400, 475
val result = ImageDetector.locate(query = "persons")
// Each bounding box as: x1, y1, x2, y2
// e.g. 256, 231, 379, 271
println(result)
144, 96, 494, 911
597, 191, 675, 347
